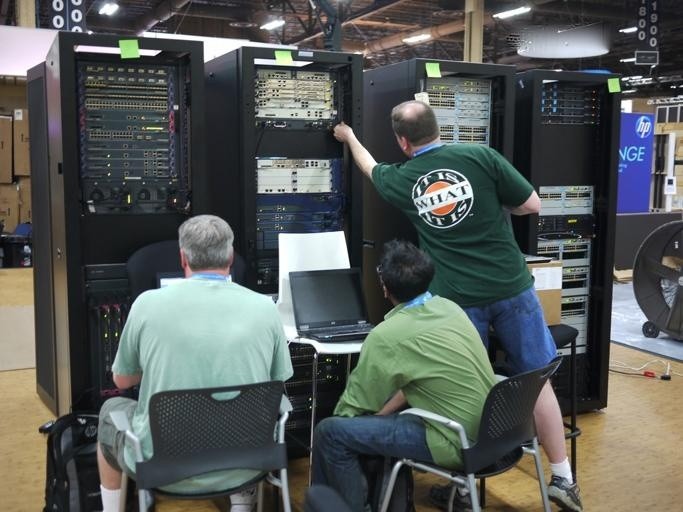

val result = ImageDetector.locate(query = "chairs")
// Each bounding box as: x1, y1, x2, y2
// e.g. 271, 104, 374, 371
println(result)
379, 358, 565, 512
106, 378, 294, 511
274, 230, 364, 491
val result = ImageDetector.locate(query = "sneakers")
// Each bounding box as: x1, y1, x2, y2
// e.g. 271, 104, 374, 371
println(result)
229, 487, 258, 512
547, 475, 583, 512
427, 480, 472, 512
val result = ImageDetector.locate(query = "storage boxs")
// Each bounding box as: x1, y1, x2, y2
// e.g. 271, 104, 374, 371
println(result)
523, 260, 562, 324
0, 108, 33, 235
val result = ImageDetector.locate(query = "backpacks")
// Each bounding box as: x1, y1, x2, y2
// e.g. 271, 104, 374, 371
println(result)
42, 411, 135, 512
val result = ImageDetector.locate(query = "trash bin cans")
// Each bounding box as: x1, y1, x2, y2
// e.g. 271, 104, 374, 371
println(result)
0, 235, 33, 268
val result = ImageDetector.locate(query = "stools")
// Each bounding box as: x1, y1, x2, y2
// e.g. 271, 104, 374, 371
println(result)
549, 325, 582, 487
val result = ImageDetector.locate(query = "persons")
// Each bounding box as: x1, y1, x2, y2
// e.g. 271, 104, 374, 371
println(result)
91, 213, 292, 511
332, 98, 584, 510
307, 234, 503, 510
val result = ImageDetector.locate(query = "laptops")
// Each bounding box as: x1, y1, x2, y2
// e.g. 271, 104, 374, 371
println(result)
154, 271, 234, 289
289, 267, 377, 344
